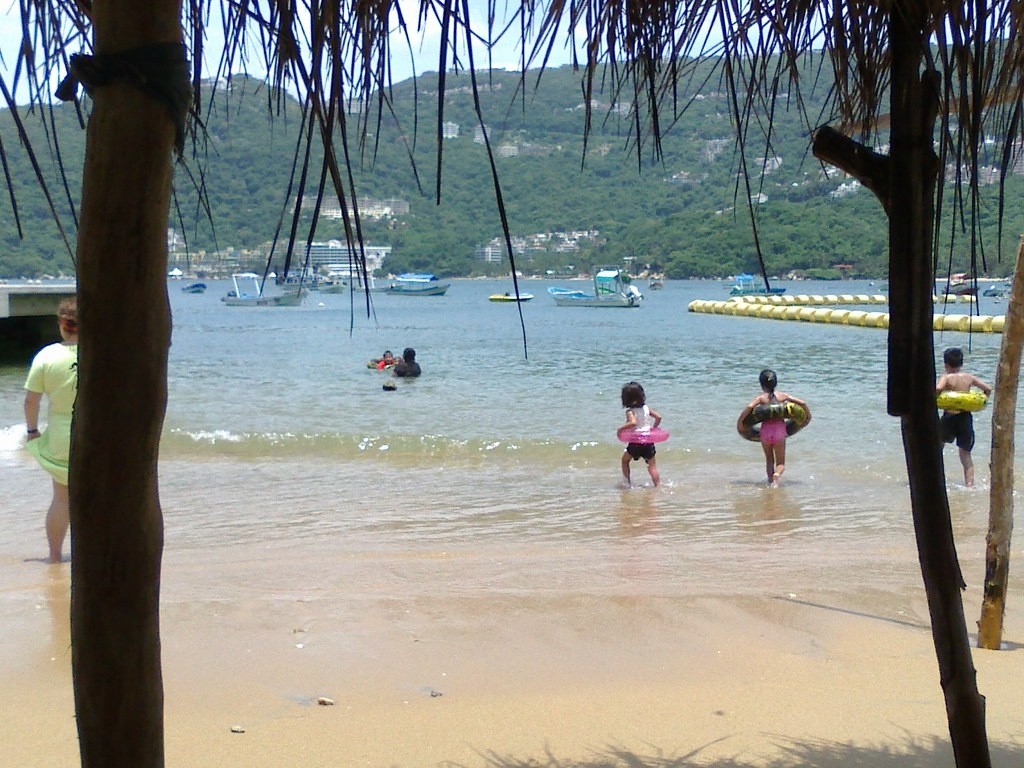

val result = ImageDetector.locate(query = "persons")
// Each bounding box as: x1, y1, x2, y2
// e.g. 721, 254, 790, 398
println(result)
24, 296, 79, 563
393, 347, 422, 378
737, 369, 811, 483
616, 381, 661, 486
377, 350, 395, 371
936, 347, 992, 487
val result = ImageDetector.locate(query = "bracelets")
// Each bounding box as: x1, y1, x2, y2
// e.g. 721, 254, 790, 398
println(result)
27, 428, 38, 434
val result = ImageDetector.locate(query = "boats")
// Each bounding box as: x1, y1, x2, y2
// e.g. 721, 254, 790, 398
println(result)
941, 271, 980, 297
182, 282, 208, 295
384, 273, 452, 297
489, 293, 537, 301
547, 269, 646, 307
352, 283, 391, 292
648, 273, 665, 291
223, 270, 344, 308
983, 287, 1014, 297
720, 271, 786, 297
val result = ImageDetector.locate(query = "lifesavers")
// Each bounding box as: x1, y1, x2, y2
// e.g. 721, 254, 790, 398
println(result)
736, 397, 810, 439
368, 356, 402, 370
931, 393, 992, 411
616, 425, 671, 443
